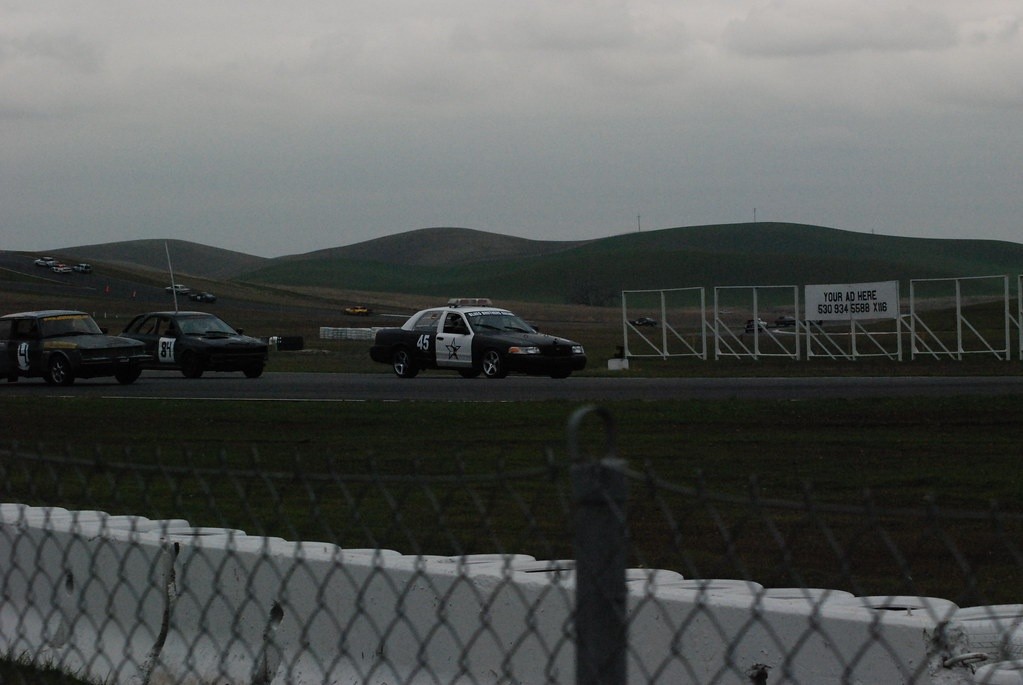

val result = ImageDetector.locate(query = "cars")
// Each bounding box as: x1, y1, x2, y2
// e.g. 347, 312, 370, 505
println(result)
188, 292, 215, 303
165, 284, 190, 295
117, 310, 268, 380
0, 309, 146, 388
345, 306, 369, 316
34, 257, 56, 267
51, 264, 72, 272
370, 306, 587, 379
630, 317, 657, 327
775, 316, 795, 326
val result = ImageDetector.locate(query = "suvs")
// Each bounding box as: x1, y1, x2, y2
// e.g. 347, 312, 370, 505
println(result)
73, 263, 92, 272
745, 318, 768, 332
